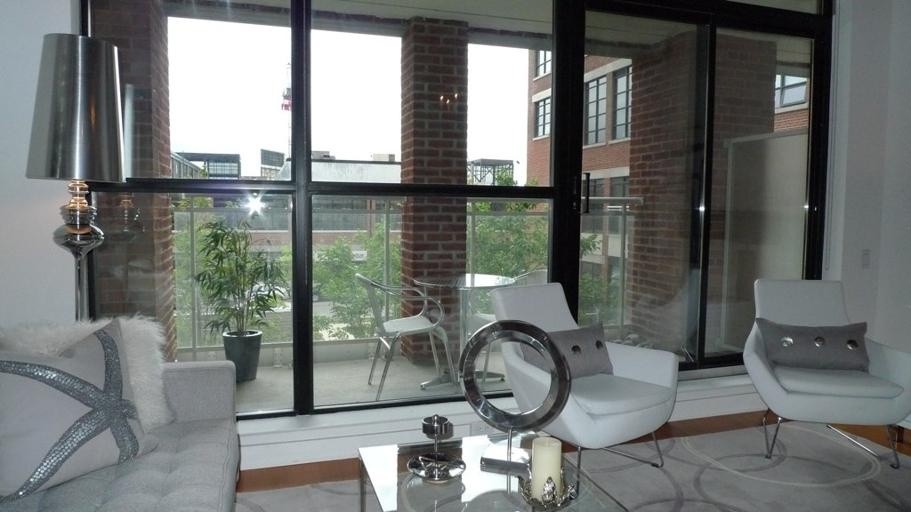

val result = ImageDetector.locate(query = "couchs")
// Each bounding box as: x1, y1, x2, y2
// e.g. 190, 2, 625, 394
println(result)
0, 312, 241, 512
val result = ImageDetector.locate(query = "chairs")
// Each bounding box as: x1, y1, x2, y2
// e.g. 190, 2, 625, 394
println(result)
742, 278, 911, 470
474, 269, 548, 383
355, 272, 457, 402
490, 282, 680, 500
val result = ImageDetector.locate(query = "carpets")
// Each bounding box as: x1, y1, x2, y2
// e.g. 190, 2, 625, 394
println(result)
235, 419, 911, 512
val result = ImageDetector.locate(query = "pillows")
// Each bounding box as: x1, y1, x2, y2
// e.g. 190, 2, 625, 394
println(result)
755, 317, 870, 373
0, 312, 176, 433
0, 319, 159, 504
519, 321, 614, 379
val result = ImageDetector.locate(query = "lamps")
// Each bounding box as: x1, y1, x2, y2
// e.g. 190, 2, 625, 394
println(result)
26, 32, 123, 320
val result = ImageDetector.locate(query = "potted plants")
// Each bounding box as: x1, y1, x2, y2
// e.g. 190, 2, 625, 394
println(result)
194, 219, 287, 383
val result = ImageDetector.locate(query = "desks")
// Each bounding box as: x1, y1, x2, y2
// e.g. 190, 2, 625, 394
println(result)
414, 273, 516, 390
